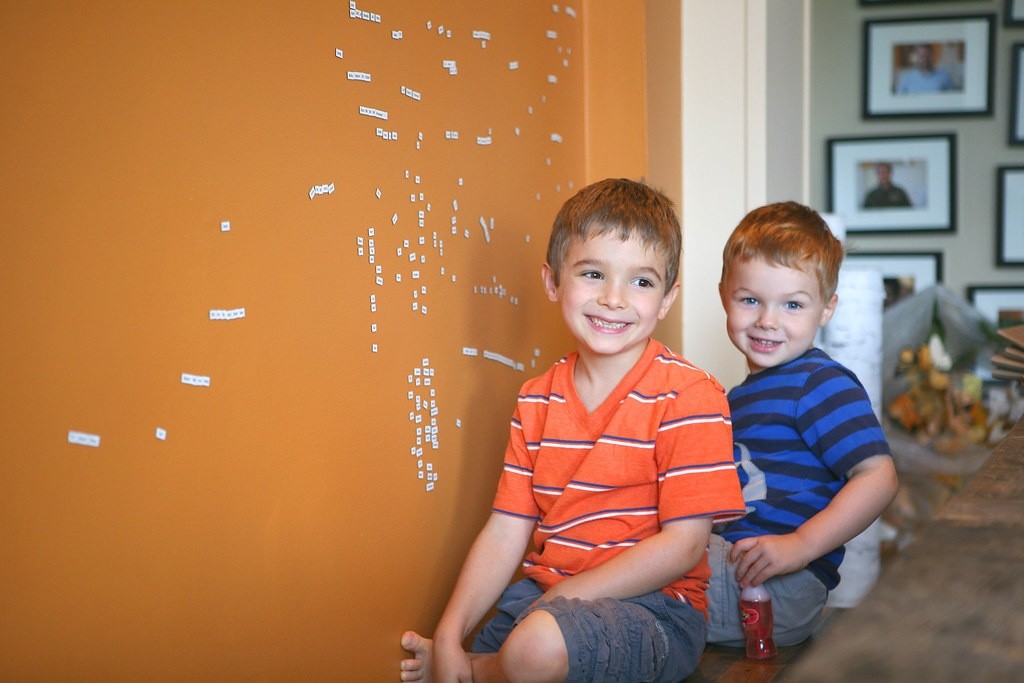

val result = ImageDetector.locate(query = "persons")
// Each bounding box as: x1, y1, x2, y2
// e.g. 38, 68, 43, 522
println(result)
861, 161, 912, 206
398, 178, 748, 683
706, 201, 901, 648
891, 44, 957, 94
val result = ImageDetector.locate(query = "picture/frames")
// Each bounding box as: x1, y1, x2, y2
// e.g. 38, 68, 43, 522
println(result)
994, 162, 1024, 268
860, 10, 994, 119
823, 130, 959, 234
966, 282, 1024, 386
1006, 38, 1024, 147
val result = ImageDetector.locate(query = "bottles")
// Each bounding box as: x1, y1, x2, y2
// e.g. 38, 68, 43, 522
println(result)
739, 550, 777, 660
